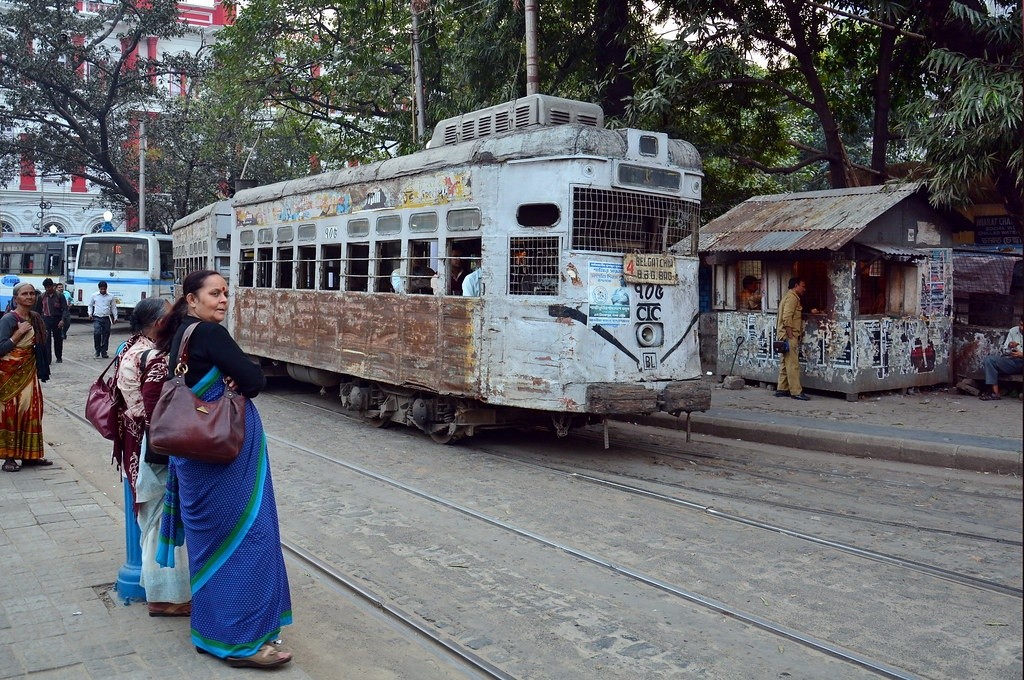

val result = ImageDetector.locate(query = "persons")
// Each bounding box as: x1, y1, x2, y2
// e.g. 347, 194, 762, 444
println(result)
980, 314, 1024, 401
0, 283, 53, 471
776, 277, 817, 400
10, 278, 74, 365
111, 296, 192, 617
87, 281, 118, 358
736, 275, 761, 310
390, 244, 481, 296
522, 250, 557, 295
156, 270, 293, 668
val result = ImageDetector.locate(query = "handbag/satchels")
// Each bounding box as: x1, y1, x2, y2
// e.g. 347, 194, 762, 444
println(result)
774, 341, 789, 353
149, 321, 245, 460
84, 353, 121, 441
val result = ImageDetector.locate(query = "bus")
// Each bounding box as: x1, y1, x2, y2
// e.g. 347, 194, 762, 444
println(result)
171, 198, 233, 307
71, 229, 176, 322
222, 89, 710, 447
0, 231, 103, 320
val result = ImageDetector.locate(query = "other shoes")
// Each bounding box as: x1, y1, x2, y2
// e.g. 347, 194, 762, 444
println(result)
94, 351, 100, 357
101, 352, 108, 358
792, 393, 811, 400
775, 389, 790, 396
55, 357, 62, 362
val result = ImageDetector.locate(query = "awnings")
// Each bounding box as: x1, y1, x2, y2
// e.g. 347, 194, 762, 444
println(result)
668, 227, 866, 251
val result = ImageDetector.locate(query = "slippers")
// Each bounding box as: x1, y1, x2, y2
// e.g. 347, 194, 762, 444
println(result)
20, 459, 53, 466
979, 393, 1000, 400
229, 644, 292, 667
1, 460, 19, 472
196, 646, 207, 653
148, 602, 193, 617
